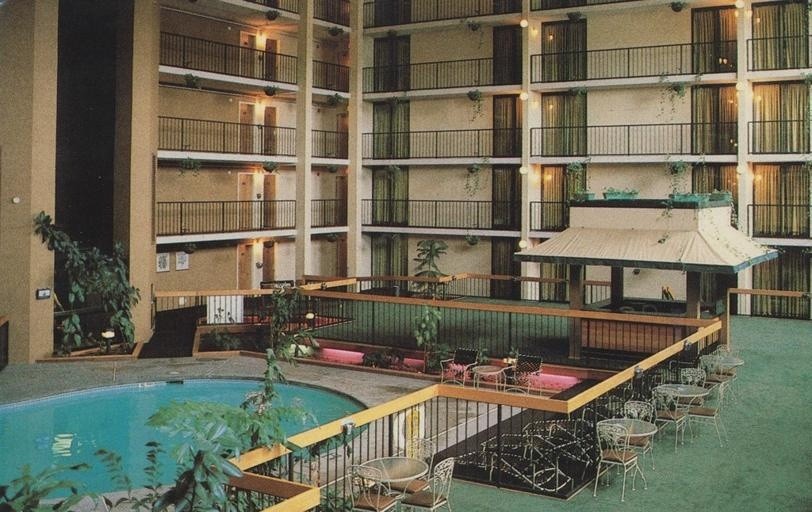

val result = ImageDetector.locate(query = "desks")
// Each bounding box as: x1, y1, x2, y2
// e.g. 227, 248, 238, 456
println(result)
356, 456, 430, 501
597, 419, 658, 480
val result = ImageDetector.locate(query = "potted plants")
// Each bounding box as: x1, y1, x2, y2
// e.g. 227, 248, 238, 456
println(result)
659, 81, 687, 120
465, 165, 490, 200
328, 27, 345, 40
326, 234, 339, 242
467, 91, 484, 122
467, 22, 481, 33
327, 94, 344, 105
262, 86, 280, 97
666, 1, 687, 13
566, 11, 582, 22
265, 10, 280, 21
325, 165, 339, 172
262, 162, 280, 174
564, 160, 732, 204
465, 235, 481, 247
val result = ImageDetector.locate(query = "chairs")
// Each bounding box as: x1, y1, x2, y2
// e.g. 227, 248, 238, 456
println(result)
651, 345, 745, 452
385, 438, 435, 495
440, 348, 479, 387
619, 401, 658, 472
620, 304, 659, 316
593, 423, 648, 502
347, 465, 398, 512
503, 355, 543, 396
454, 408, 606, 493
401, 457, 455, 512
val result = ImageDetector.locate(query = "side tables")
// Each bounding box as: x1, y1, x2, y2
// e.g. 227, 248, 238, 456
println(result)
473, 366, 504, 391
